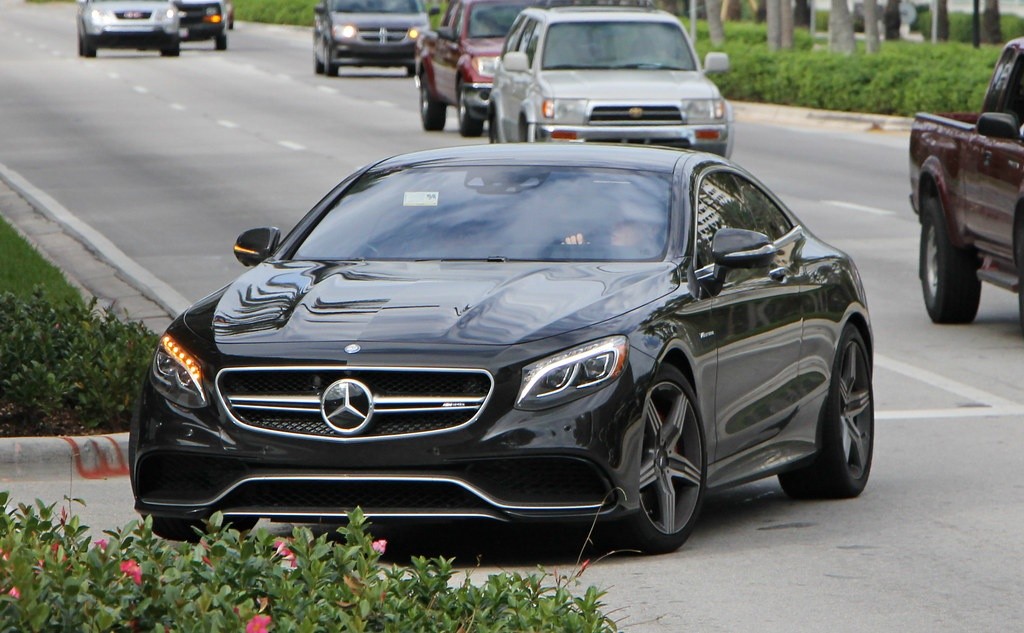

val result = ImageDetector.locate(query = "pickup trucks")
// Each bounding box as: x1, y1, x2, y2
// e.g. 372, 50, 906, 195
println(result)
907, 36, 1024, 324
414, 0, 577, 138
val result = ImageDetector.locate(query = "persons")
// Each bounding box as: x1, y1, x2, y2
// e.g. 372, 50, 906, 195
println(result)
559, 181, 664, 254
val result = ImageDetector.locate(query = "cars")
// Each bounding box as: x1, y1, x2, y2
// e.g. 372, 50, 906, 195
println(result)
127, 142, 874, 556
312, 0, 440, 77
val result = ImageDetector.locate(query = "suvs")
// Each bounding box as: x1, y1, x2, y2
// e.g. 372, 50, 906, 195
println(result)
76, 0, 183, 57
173, 0, 234, 51
488, 5, 735, 158
